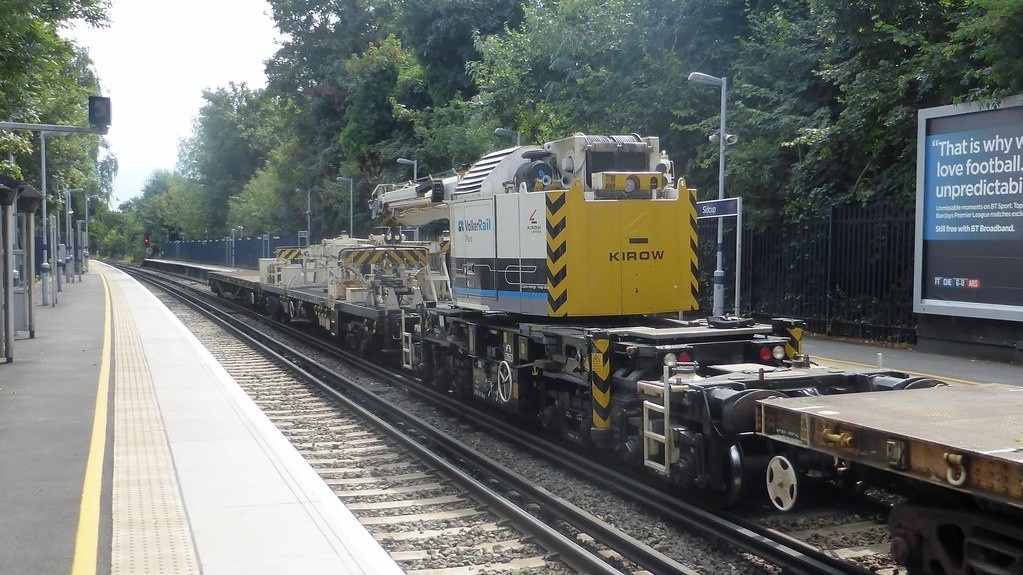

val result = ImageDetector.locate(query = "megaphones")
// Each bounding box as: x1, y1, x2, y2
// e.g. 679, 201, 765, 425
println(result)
724, 134, 738, 145
708, 129, 720, 145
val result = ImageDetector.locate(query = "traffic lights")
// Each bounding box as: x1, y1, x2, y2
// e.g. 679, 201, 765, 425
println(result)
145, 234, 150, 245
88, 95, 112, 126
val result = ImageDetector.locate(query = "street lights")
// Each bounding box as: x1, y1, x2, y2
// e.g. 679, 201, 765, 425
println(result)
495, 127, 521, 147
42, 130, 69, 309
688, 70, 728, 315
294, 185, 310, 247
68, 188, 84, 281
85, 194, 98, 273
335, 177, 353, 238
397, 158, 418, 241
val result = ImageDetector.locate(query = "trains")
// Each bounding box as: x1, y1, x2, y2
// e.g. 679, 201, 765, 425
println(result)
203, 128, 1023, 574
